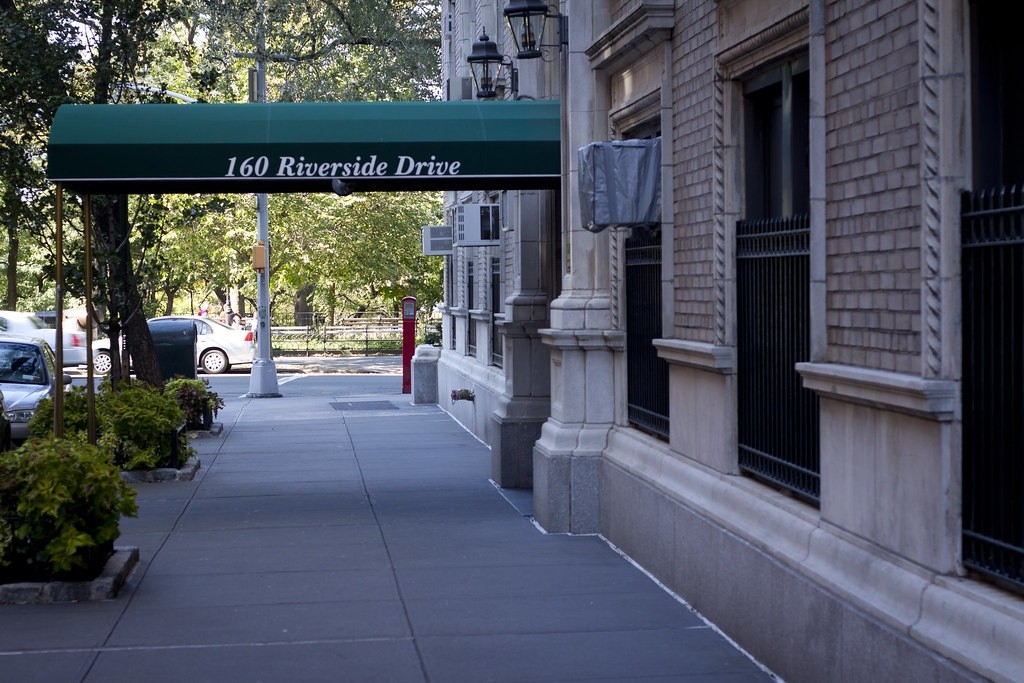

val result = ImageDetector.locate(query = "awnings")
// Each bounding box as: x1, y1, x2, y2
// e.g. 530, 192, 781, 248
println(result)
46, 99, 560, 444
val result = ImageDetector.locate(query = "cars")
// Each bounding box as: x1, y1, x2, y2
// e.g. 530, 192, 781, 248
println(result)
0, 337, 72, 440
0, 311, 92, 366
92, 315, 254, 375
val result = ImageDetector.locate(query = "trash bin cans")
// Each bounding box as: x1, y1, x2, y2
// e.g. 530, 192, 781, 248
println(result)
147, 319, 199, 384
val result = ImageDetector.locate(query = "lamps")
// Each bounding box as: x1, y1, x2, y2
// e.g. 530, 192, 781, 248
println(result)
466, 26, 534, 102
503, 0, 569, 61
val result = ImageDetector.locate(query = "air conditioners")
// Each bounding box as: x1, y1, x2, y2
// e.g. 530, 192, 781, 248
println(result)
449, 204, 500, 247
419, 225, 453, 255
579, 139, 664, 229
